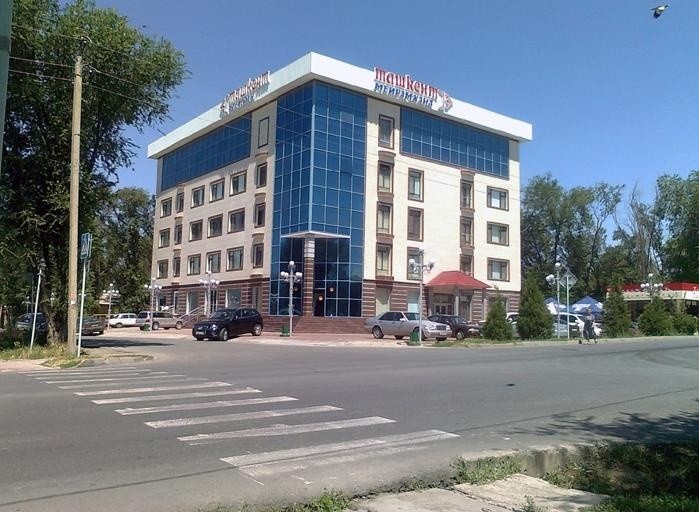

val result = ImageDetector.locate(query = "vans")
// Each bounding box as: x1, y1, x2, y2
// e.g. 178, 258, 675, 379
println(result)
135, 310, 184, 330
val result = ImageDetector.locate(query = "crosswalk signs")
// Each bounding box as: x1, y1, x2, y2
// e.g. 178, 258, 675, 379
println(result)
80, 233, 90, 260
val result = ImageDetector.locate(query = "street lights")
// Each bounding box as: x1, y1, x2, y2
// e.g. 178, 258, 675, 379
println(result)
280, 259, 304, 339
29, 257, 47, 351
101, 269, 221, 330
545, 262, 561, 339
408, 248, 435, 342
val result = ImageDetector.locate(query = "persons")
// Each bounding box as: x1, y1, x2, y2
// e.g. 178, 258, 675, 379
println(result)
582, 308, 598, 345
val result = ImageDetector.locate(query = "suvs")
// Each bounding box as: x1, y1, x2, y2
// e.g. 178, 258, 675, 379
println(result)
192, 306, 264, 341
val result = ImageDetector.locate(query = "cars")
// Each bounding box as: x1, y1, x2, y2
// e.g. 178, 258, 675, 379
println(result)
363, 310, 453, 341
106, 313, 137, 328
13, 312, 105, 337
427, 314, 480, 339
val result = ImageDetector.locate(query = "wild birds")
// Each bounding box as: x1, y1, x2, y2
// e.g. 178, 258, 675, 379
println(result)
649, 4, 669, 19
